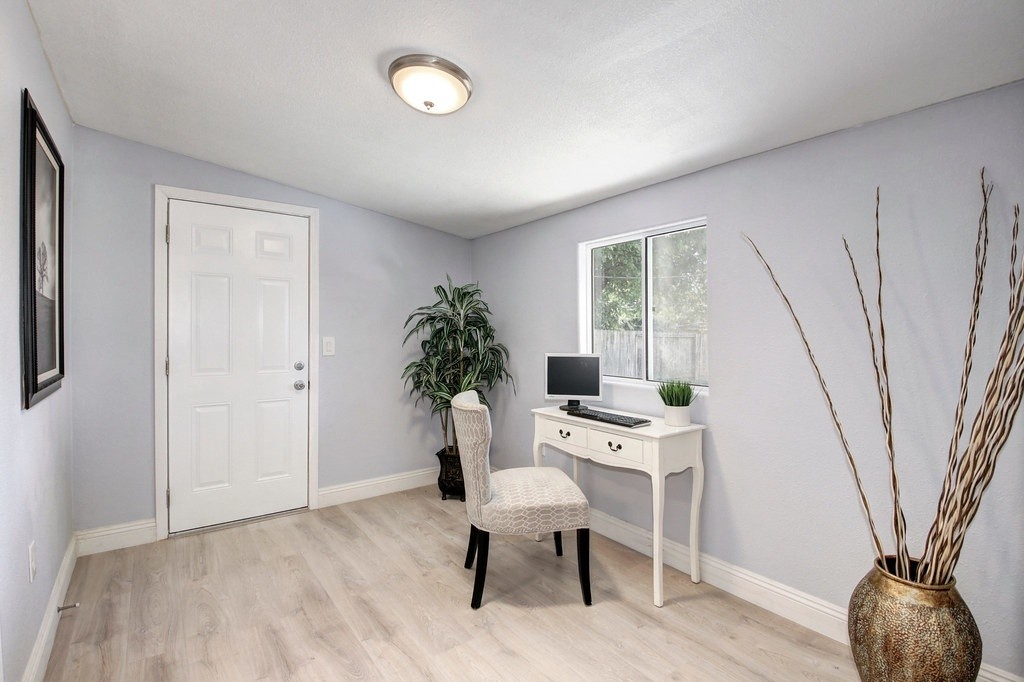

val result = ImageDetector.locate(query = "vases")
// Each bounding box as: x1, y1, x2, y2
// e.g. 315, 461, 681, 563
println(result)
848, 555, 983, 682
656, 381, 703, 426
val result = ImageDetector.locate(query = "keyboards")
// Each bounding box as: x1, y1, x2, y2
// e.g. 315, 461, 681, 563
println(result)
567, 409, 652, 429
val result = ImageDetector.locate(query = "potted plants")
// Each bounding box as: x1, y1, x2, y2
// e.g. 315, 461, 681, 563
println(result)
399, 273, 518, 501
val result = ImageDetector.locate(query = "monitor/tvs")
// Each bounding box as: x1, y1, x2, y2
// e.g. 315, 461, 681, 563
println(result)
544, 353, 602, 412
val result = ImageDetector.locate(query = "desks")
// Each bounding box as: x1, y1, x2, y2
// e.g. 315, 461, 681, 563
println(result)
531, 403, 708, 607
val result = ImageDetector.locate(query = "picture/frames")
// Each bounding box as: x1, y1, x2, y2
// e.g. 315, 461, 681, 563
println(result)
24, 87, 65, 410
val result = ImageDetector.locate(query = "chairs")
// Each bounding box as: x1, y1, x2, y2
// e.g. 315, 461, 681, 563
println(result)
451, 390, 592, 610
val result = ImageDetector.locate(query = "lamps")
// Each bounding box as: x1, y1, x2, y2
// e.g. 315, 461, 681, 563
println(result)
388, 54, 473, 117
